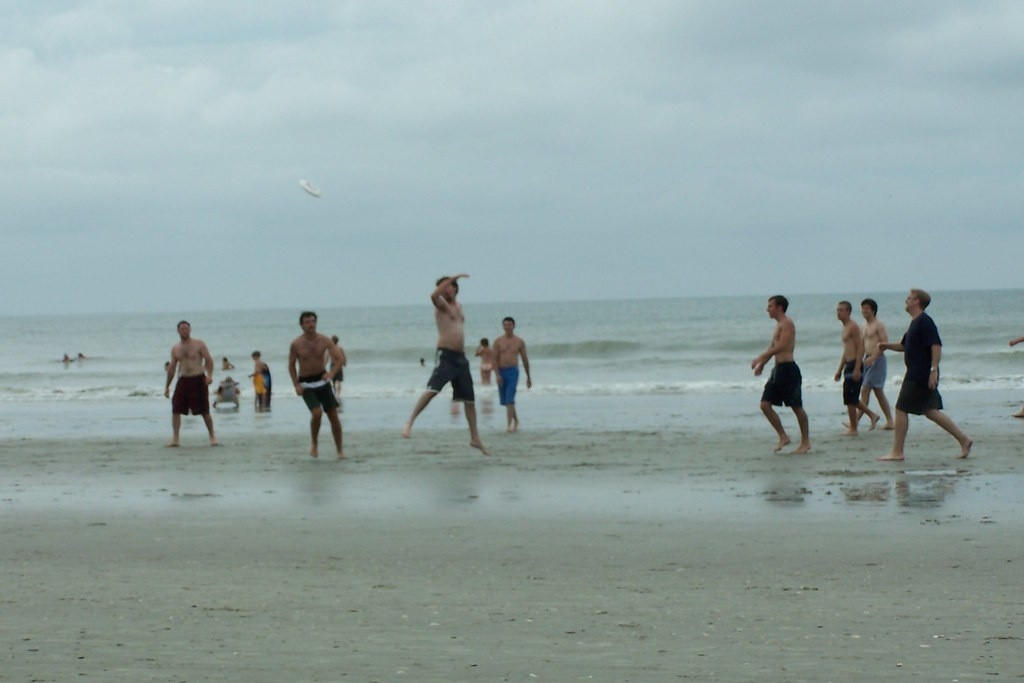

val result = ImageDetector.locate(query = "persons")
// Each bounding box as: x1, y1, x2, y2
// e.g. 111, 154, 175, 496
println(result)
63, 353, 84, 361
842, 299, 895, 429
491, 318, 531, 432
165, 362, 170, 369
212, 376, 241, 408
476, 339, 492, 384
1010, 337, 1024, 417
419, 358, 424, 366
221, 357, 234, 370
325, 335, 346, 397
164, 321, 218, 447
249, 351, 271, 407
878, 289, 973, 459
403, 274, 491, 455
835, 301, 880, 436
752, 295, 811, 453
254, 368, 266, 410
290, 312, 348, 458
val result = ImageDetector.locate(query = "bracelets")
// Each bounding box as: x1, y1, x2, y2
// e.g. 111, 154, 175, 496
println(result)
930, 368, 936, 371
